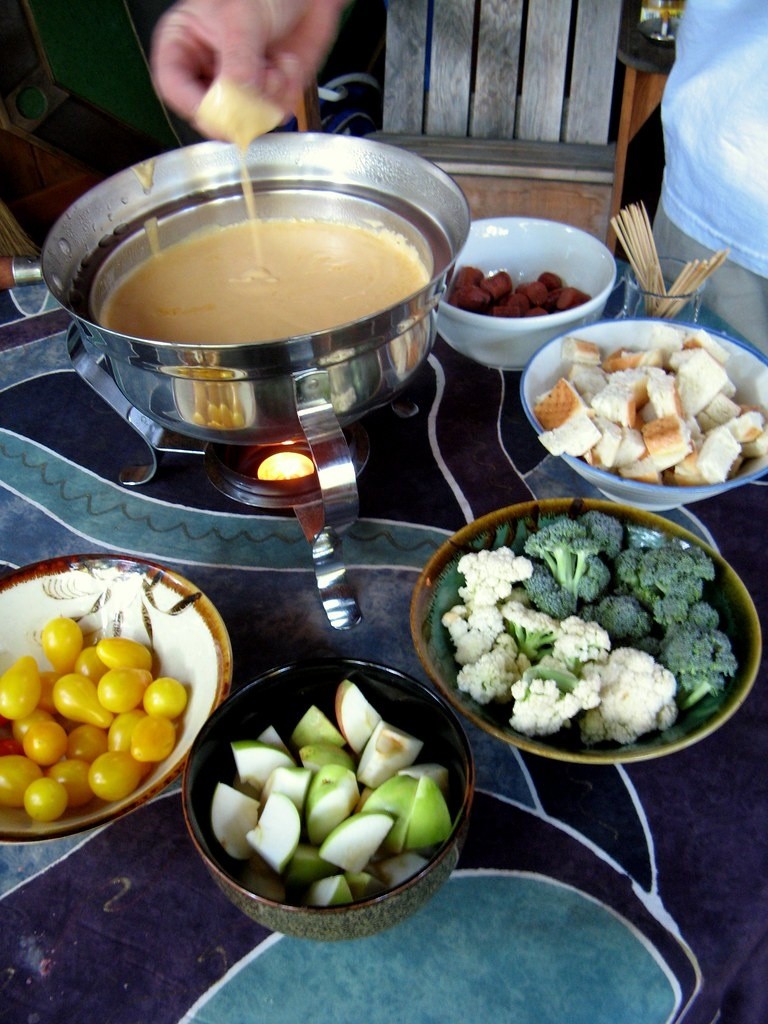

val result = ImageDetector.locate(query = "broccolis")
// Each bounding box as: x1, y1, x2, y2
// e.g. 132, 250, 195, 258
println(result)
440, 511, 737, 747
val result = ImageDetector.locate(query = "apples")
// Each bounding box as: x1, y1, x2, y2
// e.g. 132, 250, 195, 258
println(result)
209, 679, 453, 908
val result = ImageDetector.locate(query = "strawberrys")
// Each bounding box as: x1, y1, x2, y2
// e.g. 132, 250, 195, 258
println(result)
446, 267, 593, 319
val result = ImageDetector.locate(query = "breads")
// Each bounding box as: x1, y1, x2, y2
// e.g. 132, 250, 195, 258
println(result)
535, 328, 768, 486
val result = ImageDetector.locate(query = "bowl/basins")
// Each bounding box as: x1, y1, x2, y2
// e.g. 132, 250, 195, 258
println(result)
182, 657, 476, 941
0, 555, 233, 841
411, 497, 760, 762
435, 217, 616, 371
520, 319, 768, 512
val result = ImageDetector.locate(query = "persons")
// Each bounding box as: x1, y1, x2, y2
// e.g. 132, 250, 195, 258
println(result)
149, 0, 768, 362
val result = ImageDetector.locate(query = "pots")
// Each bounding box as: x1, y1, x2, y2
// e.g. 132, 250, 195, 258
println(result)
0, 133, 469, 443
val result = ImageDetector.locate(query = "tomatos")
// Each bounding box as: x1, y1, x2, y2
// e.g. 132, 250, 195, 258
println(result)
0, 617, 186, 822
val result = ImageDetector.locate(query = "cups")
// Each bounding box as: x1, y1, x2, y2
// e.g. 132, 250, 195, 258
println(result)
623, 257, 705, 325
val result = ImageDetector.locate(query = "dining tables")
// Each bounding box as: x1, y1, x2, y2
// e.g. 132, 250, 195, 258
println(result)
0, 254, 768, 1024
